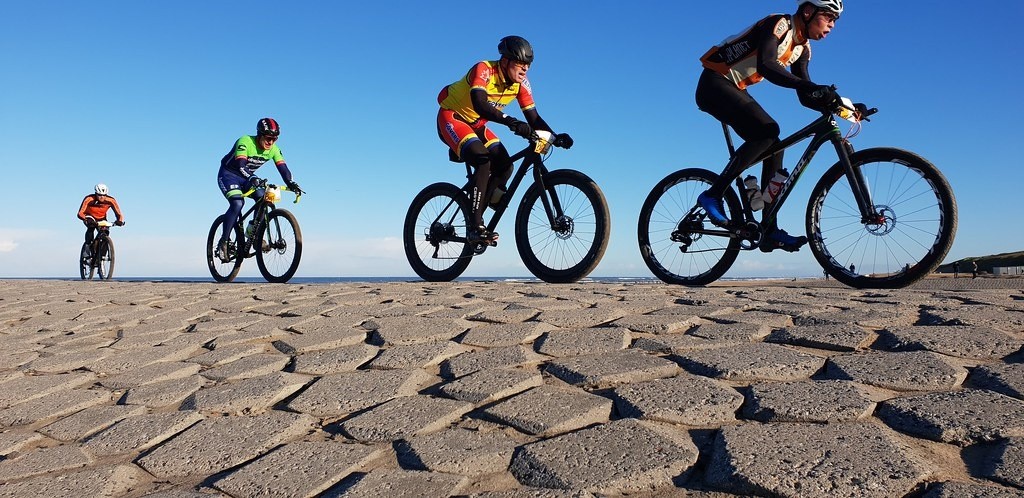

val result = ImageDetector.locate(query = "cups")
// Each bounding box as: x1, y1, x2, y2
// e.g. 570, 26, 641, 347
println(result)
245, 220, 254, 238
489, 185, 507, 208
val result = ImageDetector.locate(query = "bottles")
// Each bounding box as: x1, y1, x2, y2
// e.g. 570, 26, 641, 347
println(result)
744, 174, 764, 211
761, 168, 789, 204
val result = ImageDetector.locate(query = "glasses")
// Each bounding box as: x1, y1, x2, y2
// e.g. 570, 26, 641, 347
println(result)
817, 13, 838, 23
263, 135, 277, 141
514, 60, 525, 66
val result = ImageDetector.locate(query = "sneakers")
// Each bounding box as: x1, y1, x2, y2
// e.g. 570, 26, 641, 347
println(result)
467, 225, 499, 242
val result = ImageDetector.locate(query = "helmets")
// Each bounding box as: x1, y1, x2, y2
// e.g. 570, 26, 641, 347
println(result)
798, 0, 843, 16
94, 184, 109, 195
257, 118, 280, 136
498, 36, 534, 64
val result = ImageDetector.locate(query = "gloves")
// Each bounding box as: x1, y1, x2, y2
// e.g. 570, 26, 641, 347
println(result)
248, 176, 262, 188
853, 103, 868, 121
288, 181, 302, 192
809, 84, 843, 112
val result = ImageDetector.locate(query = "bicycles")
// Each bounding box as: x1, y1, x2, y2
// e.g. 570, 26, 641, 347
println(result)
636, 84, 959, 291
79, 219, 126, 280
401, 128, 611, 284
206, 178, 306, 284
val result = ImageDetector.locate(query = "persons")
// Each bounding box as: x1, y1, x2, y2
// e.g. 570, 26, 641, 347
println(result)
77, 184, 123, 262
695, 0, 867, 251
217, 118, 301, 263
437, 36, 574, 241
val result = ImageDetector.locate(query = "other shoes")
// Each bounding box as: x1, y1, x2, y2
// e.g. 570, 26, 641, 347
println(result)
479, 187, 504, 211
245, 226, 267, 248
697, 191, 730, 226
218, 240, 229, 263
83, 243, 91, 257
758, 230, 807, 252
98, 266, 106, 276
